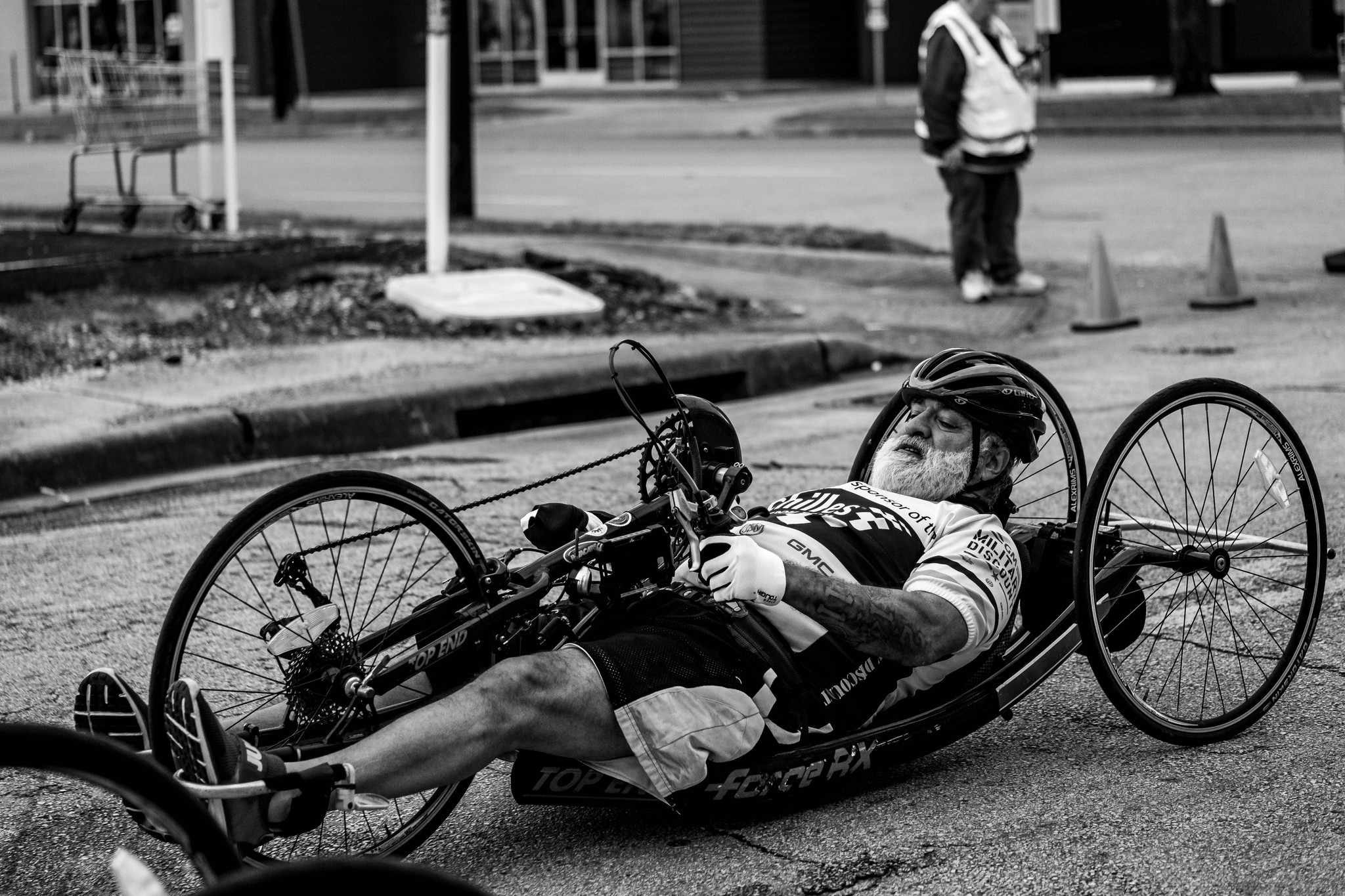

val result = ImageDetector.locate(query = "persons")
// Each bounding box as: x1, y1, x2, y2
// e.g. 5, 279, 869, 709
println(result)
162, 8, 185, 86
63, 346, 1046, 854
916, 0, 1050, 306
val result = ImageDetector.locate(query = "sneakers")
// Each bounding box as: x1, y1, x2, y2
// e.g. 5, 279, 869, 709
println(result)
74, 668, 183, 846
162, 680, 286, 860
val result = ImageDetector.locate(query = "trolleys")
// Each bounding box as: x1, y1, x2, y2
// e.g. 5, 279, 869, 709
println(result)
42, 46, 251, 233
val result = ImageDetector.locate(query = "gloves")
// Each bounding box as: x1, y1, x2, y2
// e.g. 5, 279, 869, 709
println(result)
688, 533, 785, 609
521, 503, 602, 549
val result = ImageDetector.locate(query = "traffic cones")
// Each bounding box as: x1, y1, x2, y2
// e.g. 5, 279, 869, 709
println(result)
1188, 214, 1258, 310
1069, 229, 1142, 333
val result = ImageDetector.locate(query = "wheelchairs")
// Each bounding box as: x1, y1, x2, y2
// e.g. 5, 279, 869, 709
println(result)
147, 337, 1336, 877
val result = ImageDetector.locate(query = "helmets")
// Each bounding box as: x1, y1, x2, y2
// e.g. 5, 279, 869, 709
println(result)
901, 347, 1046, 465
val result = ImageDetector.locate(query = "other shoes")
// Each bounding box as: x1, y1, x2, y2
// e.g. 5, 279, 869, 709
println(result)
960, 270, 990, 303
987, 270, 1047, 296
